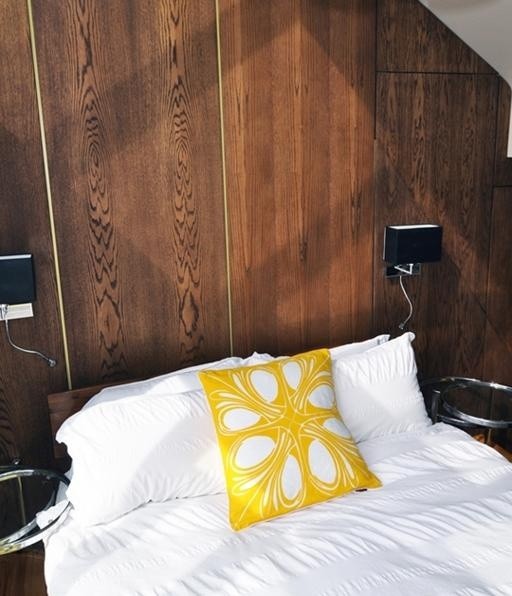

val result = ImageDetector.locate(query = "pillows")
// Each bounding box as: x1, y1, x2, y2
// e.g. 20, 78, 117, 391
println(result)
79, 357, 244, 409
197, 347, 382, 530
331, 332, 430, 446
56, 390, 229, 529
245, 331, 391, 365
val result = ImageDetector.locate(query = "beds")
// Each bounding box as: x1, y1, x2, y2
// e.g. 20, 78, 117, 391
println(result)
46, 362, 512, 596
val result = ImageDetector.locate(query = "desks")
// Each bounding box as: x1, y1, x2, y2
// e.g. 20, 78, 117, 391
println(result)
0, 460, 71, 557
420, 377, 512, 447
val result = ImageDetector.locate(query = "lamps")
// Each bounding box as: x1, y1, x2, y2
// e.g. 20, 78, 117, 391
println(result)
382, 223, 443, 279
0, 253, 34, 321
